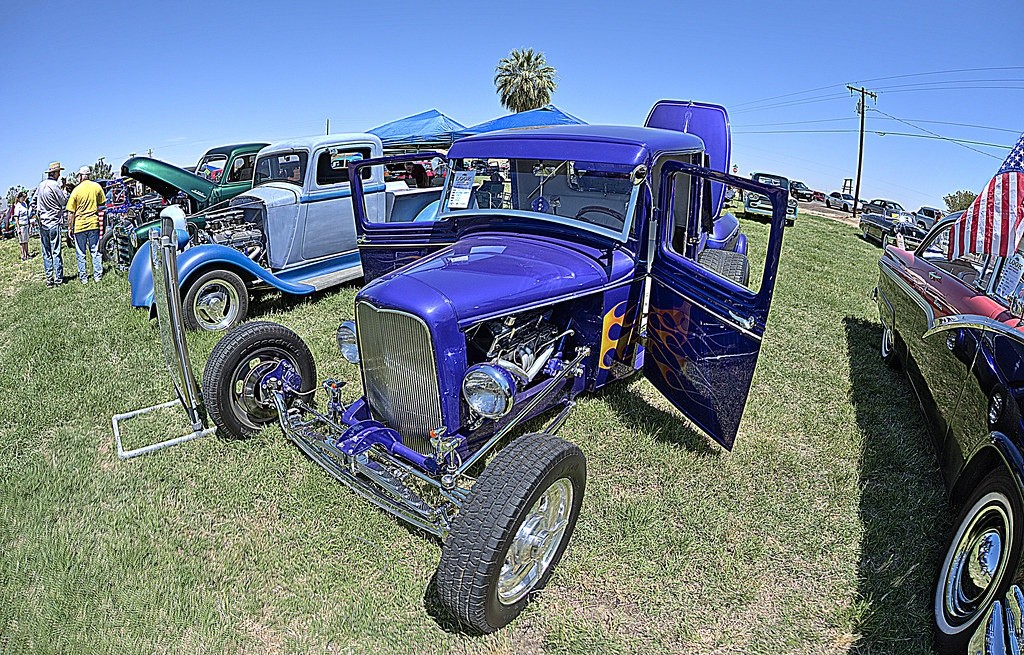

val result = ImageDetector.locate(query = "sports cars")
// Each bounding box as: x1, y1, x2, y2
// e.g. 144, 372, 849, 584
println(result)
859, 207, 944, 254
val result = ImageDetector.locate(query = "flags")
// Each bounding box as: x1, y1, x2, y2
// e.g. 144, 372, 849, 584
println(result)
948, 133, 1024, 261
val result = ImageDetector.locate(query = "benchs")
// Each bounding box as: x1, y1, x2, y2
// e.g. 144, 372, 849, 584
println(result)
539, 191, 635, 231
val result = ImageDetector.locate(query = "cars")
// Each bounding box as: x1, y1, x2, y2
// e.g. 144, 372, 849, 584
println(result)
0, 141, 510, 267
825, 192, 864, 213
873, 208, 1024, 655
862, 199, 906, 218
742, 170, 799, 228
913, 207, 951, 235
198, 100, 793, 639
727, 180, 830, 202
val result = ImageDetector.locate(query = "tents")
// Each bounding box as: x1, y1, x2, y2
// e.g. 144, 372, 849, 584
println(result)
363, 109, 467, 155
452, 102, 589, 140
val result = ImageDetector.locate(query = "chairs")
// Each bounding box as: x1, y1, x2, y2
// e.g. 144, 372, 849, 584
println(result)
240, 168, 252, 181
929, 259, 979, 286
474, 184, 511, 209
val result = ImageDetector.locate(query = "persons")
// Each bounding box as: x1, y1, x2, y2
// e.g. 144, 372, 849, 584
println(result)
29, 162, 69, 288
66, 166, 107, 284
405, 162, 429, 187
933, 210, 945, 247
106, 187, 118, 205
14, 192, 32, 260
490, 172, 505, 182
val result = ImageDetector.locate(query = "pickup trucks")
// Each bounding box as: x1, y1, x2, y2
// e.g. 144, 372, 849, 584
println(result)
130, 132, 481, 335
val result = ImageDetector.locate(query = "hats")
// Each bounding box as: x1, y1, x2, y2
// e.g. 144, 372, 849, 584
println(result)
75, 165, 92, 177
17, 192, 27, 198
44, 162, 65, 173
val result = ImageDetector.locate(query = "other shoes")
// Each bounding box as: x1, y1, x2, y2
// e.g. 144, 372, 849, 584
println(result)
46, 282, 62, 288
20, 255, 33, 261
81, 277, 102, 285
